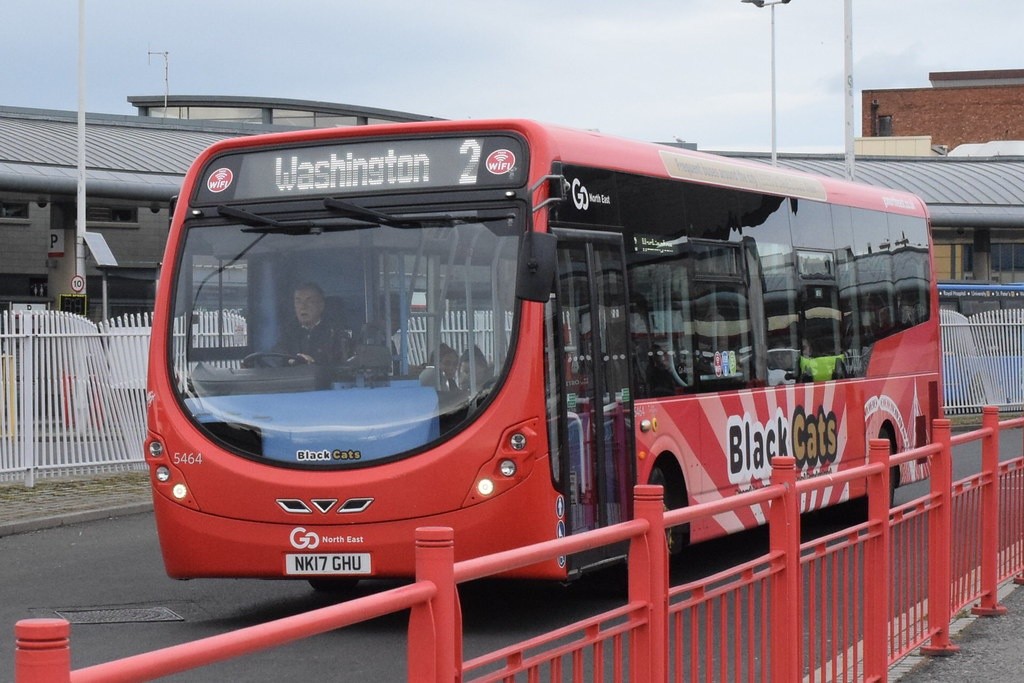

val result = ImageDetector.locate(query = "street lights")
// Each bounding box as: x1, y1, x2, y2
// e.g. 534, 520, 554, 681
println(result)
742, 0, 793, 165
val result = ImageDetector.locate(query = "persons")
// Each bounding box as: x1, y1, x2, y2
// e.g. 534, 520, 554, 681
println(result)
578, 275, 915, 398
415, 343, 487, 403
241, 282, 410, 378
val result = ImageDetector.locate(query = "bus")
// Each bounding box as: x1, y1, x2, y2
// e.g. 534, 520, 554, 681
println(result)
141, 117, 949, 634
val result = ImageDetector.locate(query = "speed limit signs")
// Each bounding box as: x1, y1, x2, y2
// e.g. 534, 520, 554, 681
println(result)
70, 276, 84, 293
